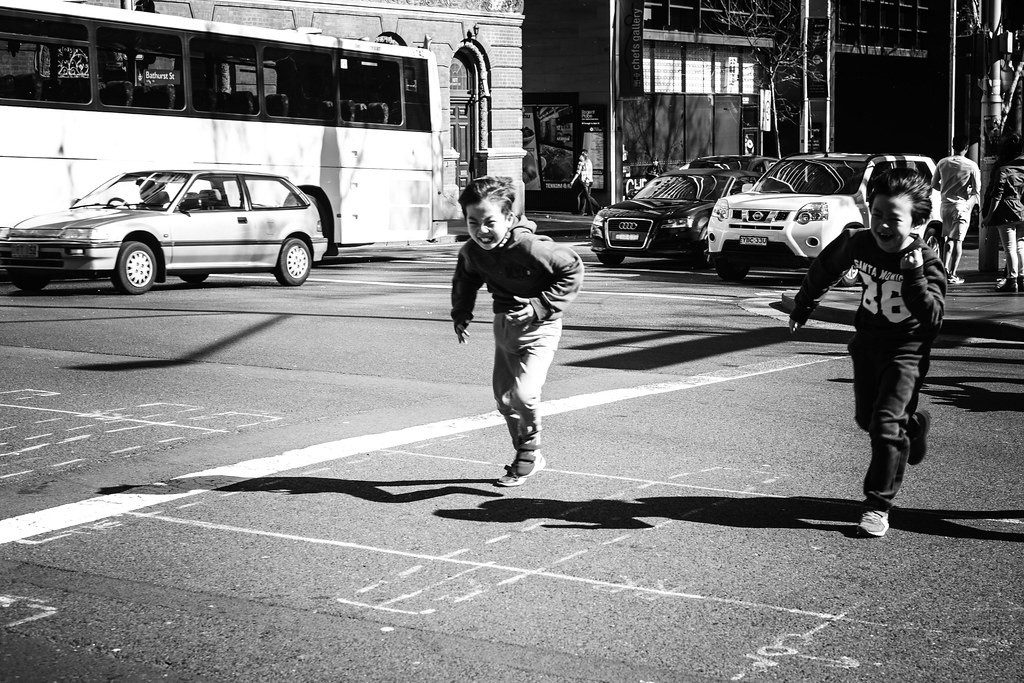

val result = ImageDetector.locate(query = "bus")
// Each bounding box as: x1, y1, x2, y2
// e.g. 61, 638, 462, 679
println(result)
0, 0, 444, 268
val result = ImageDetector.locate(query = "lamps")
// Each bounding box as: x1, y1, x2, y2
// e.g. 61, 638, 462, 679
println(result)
467, 23, 479, 40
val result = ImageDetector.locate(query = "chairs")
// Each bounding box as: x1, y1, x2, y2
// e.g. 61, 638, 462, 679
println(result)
185, 190, 222, 211
1, 72, 389, 125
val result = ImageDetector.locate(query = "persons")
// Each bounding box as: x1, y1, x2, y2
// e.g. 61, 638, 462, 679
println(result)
451, 176, 585, 486
570, 149, 603, 216
981, 133, 1024, 293
789, 167, 948, 538
932, 134, 981, 285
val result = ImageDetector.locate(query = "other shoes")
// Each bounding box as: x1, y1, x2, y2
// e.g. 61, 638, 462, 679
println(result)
995, 278, 1024, 292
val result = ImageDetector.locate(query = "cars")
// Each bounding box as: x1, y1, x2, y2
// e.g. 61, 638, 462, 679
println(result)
591, 169, 764, 271
681, 154, 779, 174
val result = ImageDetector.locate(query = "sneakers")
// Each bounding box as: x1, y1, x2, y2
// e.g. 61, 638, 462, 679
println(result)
516, 434, 540, 477
497, 454, 546, 487
858, 507, 889, 537
906, 410, 930, 465
948, 272, 965, 284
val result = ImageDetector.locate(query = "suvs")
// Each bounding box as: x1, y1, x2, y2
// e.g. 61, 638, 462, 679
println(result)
707, 152, 946, 287
0, 170, 328, 295
